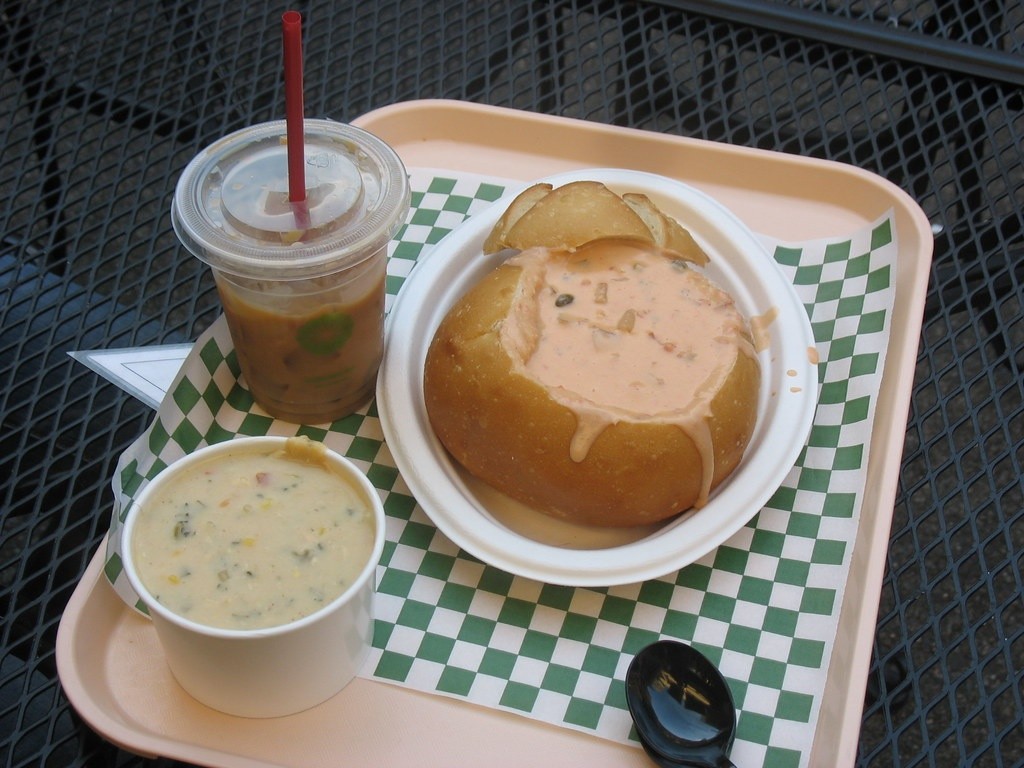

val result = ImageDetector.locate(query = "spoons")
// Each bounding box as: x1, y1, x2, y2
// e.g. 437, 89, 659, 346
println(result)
624, 639, 737, 767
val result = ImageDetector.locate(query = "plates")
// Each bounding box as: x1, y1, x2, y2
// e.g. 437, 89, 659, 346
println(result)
379, 168, 819, 584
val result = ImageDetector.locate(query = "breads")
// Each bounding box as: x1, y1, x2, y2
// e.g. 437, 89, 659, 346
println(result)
420, 178, 762, 530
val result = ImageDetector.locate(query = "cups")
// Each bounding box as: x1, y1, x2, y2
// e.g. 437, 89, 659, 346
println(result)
169, 119, 410, 424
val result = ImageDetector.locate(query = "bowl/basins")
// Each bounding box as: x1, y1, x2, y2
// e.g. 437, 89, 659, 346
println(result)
121, 434, 388, 717
